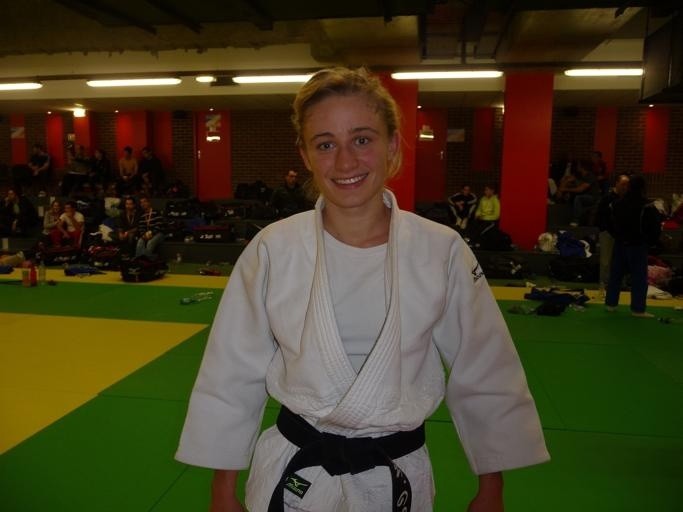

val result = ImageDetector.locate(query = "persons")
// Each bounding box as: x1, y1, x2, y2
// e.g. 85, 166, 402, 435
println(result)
1, 144, 171, 263
549, 151, 682, 317
270, 170, 310, 219
447, 185, 478, 229
467, 185, 500, 248
174, 66, 551, 511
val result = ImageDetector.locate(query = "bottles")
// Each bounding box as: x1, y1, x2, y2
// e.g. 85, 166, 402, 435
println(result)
21, 260, 46, 288
